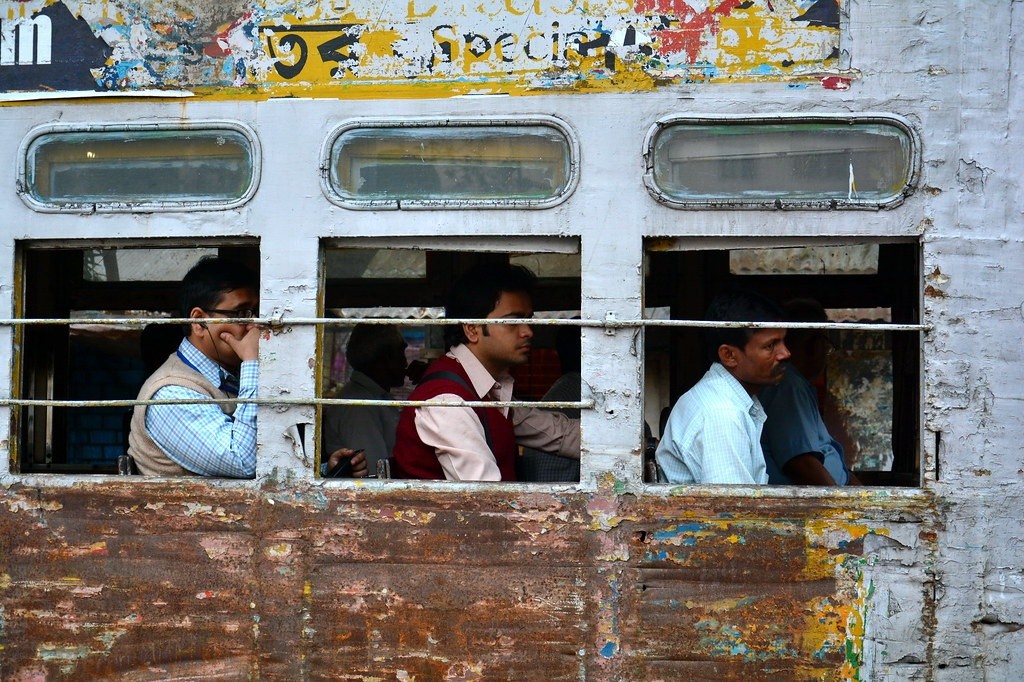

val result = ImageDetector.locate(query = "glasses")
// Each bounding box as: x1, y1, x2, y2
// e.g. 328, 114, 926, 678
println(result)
821, 332, 842, 356
202, 307, 259, 327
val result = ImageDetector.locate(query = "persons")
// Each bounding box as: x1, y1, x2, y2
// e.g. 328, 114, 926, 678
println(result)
759, 303, 862, 486
392, 262, 581, 482
654, 294, 791, 485
323, 316, 430, 475
127, 257, 369, 479
516, 371, 660, 482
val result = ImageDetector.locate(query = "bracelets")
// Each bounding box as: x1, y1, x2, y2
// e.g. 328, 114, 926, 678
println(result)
646, 435, 657, 465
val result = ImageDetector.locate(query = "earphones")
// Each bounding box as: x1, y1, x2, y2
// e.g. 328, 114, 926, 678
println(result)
199, 322, 207, 329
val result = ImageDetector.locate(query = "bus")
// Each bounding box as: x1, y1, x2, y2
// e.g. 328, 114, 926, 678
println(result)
2, 0, 1024, 682
